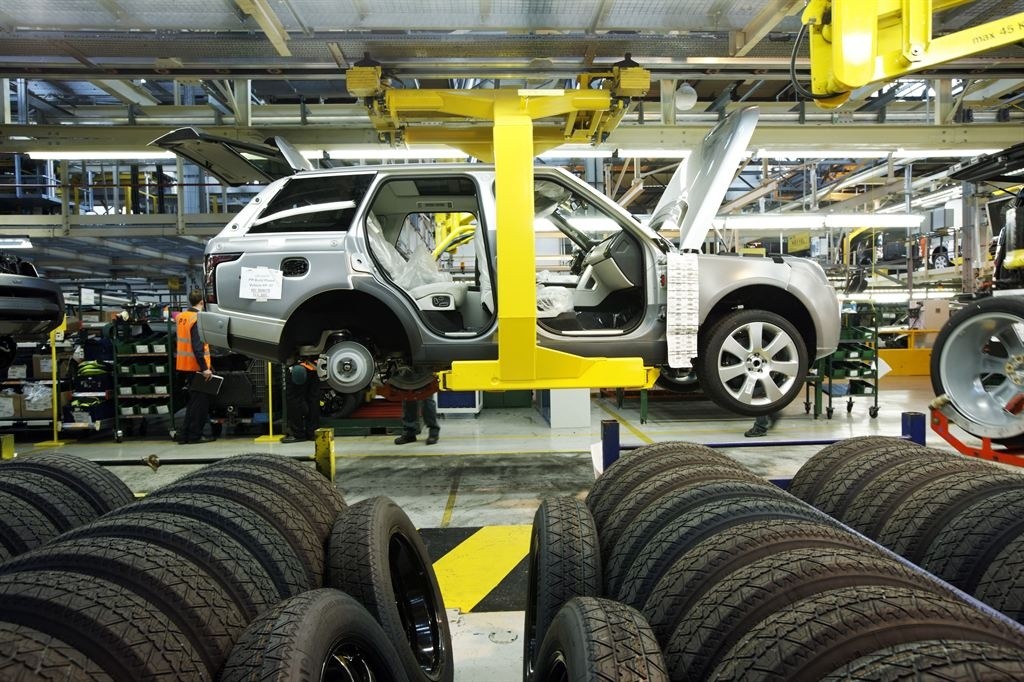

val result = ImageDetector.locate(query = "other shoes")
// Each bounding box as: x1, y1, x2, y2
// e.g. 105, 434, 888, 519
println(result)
184, 435, 216, 443
427, 435, 439, 444
395, 434, 416, 444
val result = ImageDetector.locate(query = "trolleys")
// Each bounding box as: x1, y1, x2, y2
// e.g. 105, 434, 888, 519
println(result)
803, 301, 880, 420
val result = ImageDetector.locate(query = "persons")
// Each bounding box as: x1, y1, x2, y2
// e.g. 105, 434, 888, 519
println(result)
744, 411, 782, 437
175, 291, 216, 444
395, 394, 441, 444
281, 367, 319, 443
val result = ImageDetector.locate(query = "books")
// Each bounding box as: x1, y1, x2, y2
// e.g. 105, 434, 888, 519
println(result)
190, 372, 224, 394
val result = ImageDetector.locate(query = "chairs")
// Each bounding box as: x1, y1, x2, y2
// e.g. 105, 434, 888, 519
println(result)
368, 210, 468, 311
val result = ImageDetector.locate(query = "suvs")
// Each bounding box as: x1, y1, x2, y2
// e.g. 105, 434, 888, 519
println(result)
144, 104, 842, 415
850, 229, 907, 265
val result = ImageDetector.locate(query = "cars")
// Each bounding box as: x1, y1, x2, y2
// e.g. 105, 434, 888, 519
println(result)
913, 227, 964, 270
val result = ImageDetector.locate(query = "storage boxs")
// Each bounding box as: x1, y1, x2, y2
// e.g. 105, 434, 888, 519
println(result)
0, 394, 20, 419
32, 354, 70, 379
21, 393, 63, 419
